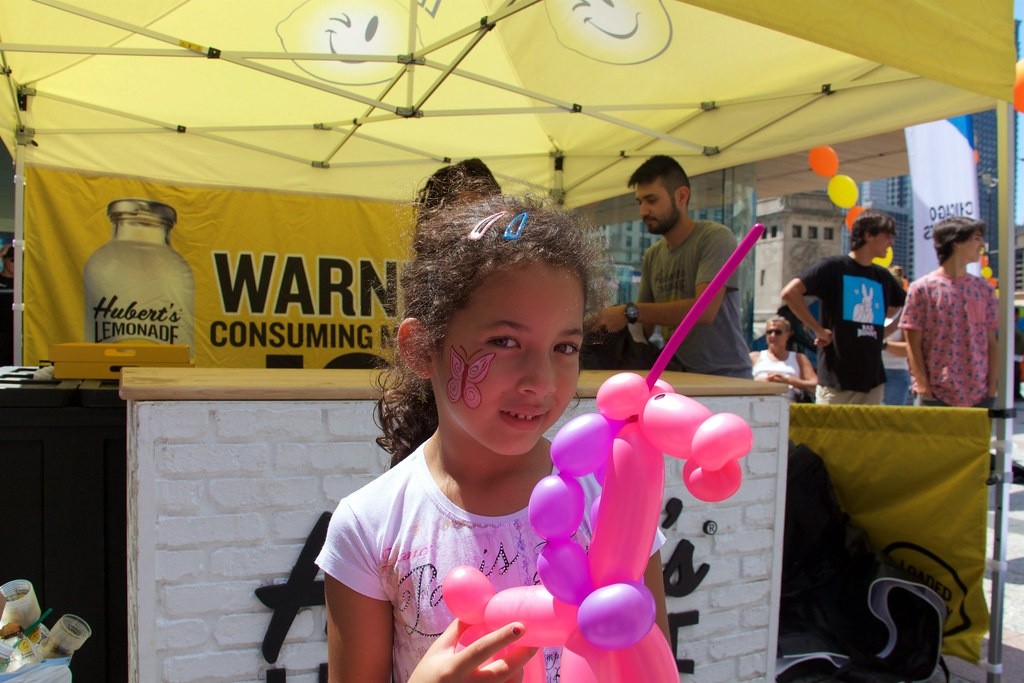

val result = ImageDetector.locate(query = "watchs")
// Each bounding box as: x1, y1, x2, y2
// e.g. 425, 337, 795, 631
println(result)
624, 302, 639, 324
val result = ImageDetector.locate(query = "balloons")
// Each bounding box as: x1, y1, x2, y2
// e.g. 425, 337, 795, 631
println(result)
1013, 58, 1024, 116
810, 145, 1007, 321
438, 221, 768, 682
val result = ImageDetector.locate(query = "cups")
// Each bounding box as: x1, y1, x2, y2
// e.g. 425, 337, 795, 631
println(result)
0, 579, 92, 671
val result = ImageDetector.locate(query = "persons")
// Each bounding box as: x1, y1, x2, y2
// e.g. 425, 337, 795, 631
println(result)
586, 152, 756, 380
902, 216, 1002, 410
780, 209, 909, 405
747, 316, 818, 402
310, 158, 674, 683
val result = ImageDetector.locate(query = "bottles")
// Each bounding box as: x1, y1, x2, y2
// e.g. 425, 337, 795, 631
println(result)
79, 197, 197, 355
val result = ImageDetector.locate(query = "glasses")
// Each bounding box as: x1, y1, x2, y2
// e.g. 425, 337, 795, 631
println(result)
766, 329, 785, 336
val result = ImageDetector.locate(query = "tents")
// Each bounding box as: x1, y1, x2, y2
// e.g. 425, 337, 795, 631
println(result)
4, 0, 1016, 683
